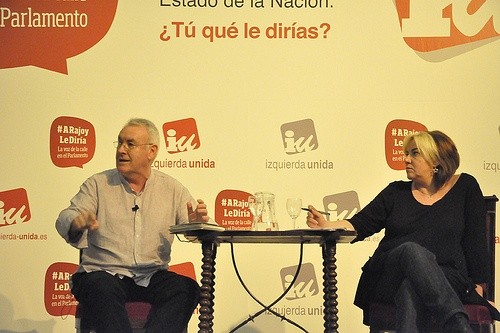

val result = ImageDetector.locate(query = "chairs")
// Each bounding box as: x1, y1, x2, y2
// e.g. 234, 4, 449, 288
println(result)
76, 248, 154, 333
369, 195, 496, 333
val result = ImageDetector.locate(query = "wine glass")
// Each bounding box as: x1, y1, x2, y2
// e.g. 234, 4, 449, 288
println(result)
248, 196, 264, 231
286, 197, 302, 230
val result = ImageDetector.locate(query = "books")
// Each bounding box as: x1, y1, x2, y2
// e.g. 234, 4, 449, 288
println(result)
169, 222, 225, 234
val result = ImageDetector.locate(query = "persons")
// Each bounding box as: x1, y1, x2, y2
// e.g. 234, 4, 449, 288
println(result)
306, 131, 500, 333
55, 119, 209, 333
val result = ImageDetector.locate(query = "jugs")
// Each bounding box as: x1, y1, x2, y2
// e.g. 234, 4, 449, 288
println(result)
251, 192, 278, 231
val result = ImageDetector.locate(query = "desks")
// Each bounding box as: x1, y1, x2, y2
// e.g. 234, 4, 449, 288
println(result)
183, 228, 357, 333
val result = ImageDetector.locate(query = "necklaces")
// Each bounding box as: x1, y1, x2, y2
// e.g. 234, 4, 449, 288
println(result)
415, 182, 437, 198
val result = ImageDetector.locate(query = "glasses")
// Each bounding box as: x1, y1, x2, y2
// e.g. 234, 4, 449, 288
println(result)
112, 140, 152, 150
